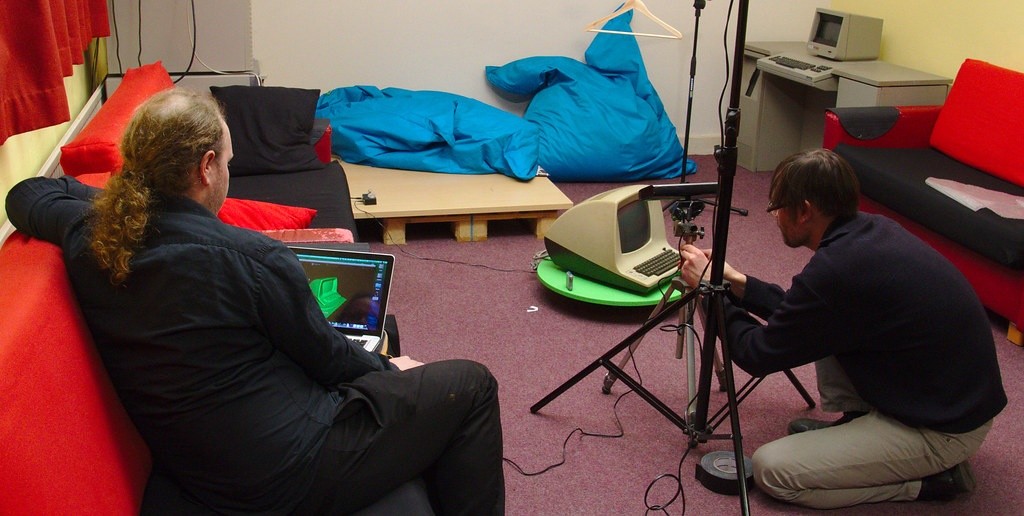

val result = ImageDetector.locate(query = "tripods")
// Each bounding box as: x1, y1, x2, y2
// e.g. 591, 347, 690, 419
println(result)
530, 0, 816, 516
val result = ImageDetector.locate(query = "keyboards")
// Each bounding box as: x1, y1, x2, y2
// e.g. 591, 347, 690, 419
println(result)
757, 53, 837, 83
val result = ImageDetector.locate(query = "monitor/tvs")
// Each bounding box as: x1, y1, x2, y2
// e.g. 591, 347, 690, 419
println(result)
807, 8, 883, 61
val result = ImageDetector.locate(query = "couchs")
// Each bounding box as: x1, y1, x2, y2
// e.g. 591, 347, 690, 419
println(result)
823, 58, 1024, 347
0, 71, 430, 516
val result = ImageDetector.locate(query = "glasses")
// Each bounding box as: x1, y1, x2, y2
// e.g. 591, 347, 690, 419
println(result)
766, 198, 800, 217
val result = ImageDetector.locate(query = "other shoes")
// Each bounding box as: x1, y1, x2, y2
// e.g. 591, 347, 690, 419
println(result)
926, 460, 976, 500
788, 414, 857, 434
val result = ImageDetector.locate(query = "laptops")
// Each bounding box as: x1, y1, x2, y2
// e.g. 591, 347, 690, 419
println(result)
286, 246, 395, 352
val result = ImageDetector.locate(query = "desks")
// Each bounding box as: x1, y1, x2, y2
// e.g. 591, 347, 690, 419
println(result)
735, 41, 953, 173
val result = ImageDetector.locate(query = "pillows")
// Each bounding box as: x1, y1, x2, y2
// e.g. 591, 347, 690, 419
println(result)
209, 85, 326, 175
217, 198, 318, 231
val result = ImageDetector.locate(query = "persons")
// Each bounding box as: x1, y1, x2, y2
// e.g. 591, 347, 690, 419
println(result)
6, 82, 505, 516
336, 290, 380, 331
679, 147, 1007, 509
